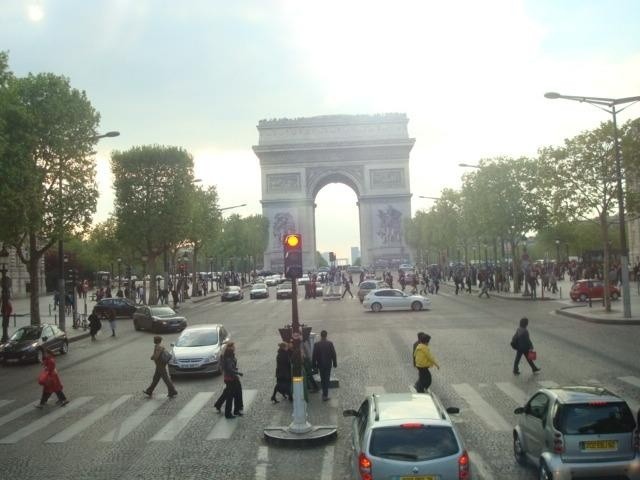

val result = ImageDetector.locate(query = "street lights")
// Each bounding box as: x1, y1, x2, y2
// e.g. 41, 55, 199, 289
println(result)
117, 256, 123, 298
218, 203, 248, 213
206, 253, 217, 293
192, 178, 204, 183
543, 87, 640, 319
110, 258, 114, 282
177, 253, 191, 301
520, 234, 532, 297
59, 129, 121, 329
458, 159, 485, 171
416, 194, 445, 202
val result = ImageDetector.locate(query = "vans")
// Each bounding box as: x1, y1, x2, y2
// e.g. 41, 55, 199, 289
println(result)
339, 389, 472, 480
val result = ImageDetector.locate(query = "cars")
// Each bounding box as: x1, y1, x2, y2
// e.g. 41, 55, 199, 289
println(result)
347, 264, 362, 273
0, 324, 70, 368
263, 266, 329, 299
167, 324, 235, 379
221, 285, 243, 300
91, 297, 148, 319
132, 303, 188, 334
250, 283, 269, 298
361, 287, 432, 313
358, 281, 392, 303
395, 257, 512, 272
507, 382, 640, 479
570, 279, 621, 302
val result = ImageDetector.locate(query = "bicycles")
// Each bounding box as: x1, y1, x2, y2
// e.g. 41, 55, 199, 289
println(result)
73, 312, 89, 331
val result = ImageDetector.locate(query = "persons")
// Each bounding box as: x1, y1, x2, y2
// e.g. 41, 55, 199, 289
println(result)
1, 269, 272, 342
33, 348, 69, 409
142, 336, 178, 396
413, 332, 439, 393
305, 264, 640, 299
513, 318, 542, 374
214, 330, 337, 418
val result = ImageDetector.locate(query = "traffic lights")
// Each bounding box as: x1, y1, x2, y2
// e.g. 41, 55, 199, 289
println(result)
282, 234, 303, 278
328, 251, 336, 262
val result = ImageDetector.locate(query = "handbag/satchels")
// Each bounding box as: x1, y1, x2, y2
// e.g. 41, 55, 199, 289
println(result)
511, 334, 518, 349
528, 351, 536, 360
38, 371, 48, 385
